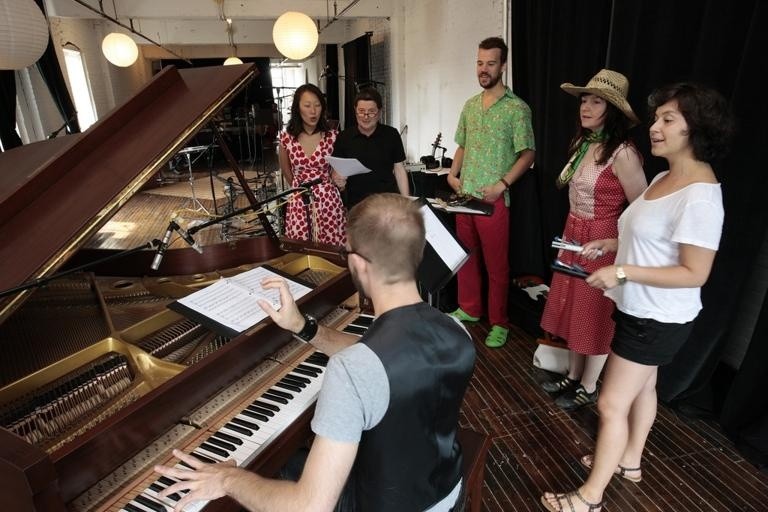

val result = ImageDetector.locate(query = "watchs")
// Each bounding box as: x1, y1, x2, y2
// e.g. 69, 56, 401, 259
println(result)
616, 264, 627, 286
292, 313, 318, 346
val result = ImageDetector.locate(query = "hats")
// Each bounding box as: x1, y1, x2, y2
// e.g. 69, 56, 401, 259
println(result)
557, 67, 639, 122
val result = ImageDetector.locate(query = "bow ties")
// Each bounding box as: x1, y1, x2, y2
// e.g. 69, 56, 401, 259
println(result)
556, 129, 616, 188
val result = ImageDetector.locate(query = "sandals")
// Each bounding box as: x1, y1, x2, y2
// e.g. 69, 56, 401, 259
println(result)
539, 488, 606, 512
582, 452, 642, 482
541, 375, 600, 410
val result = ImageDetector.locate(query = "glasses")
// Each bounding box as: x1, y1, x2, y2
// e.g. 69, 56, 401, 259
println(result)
355, 111, 379, 118
338, 247, 372, 265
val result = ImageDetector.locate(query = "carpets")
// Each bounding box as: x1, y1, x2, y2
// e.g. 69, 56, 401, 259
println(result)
143, 167, 280, 200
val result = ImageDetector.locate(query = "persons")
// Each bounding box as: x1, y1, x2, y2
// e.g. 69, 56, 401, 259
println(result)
541, 69, 648, 410
278, 83, 351, 248
154, 191, 478, 511
331, 88, 410, 212
540, 81, 738, 512
446, 35, 536, 348
269, 103, 282, 137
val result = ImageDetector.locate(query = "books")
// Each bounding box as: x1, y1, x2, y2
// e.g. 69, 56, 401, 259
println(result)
407, 195, 472, 294
165, 264, 317, 340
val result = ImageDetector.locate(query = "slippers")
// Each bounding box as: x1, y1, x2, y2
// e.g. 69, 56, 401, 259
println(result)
445, 309, 481, 325
485, 324, 508, 349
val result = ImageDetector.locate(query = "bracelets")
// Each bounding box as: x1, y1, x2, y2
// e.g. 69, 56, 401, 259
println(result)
500, 178, 511, 189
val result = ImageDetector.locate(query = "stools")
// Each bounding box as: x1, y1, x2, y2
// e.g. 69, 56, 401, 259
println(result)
451, 424, 494, 512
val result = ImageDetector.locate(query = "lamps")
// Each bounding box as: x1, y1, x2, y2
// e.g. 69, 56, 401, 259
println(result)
0, 1, 49, 72
271, 11, 319, 62
100, 20, 139, 69
223, 28, 242, 66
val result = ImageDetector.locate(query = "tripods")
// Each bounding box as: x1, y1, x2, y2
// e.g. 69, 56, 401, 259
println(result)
175, 165, 210, 217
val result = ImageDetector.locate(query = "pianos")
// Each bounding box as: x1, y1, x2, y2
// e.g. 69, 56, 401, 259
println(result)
408, 196, 493, 215
0, 64, 379, 510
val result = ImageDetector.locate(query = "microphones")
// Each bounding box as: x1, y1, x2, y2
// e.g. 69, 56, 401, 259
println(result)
171, 222, 204, 255
151, 225, 171, 271
